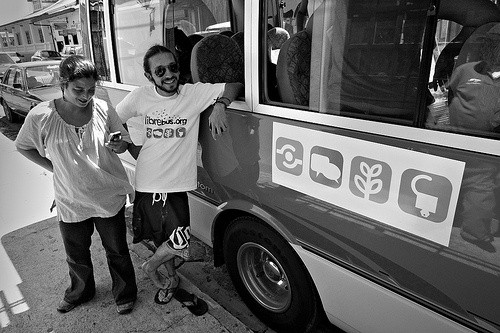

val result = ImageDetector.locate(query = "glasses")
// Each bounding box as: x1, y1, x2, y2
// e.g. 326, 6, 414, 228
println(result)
154, 63, 180, 77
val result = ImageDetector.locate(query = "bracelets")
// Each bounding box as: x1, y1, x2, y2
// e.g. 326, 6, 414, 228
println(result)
212, 96, 231, 111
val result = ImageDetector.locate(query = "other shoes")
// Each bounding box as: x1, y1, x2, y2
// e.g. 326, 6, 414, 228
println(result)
117, 301, 134, 314
56, 299, 76, 312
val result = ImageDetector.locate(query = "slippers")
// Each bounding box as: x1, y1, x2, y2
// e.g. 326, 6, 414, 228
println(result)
154, 275, 180, 305
173, 287, 208, 316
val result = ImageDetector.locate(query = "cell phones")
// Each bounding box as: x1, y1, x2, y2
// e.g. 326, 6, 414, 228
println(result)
108, 130, 121, 141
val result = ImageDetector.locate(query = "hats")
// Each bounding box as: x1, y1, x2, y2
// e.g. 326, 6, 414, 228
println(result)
478, 23, 500, 40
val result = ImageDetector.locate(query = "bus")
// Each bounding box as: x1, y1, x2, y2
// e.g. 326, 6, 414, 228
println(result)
79, 1, 500, 333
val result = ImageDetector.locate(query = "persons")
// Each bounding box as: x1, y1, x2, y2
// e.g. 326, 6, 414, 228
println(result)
445, 22, 500, 131
15, 55, 137, 314
304, 0, 500, 126
115, 44, 243, 305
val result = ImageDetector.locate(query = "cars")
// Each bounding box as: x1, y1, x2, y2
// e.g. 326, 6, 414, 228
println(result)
7, 52, 24, 64
0, 52, 19, 78
30, 50, 62, 60
0, 60, 64, 121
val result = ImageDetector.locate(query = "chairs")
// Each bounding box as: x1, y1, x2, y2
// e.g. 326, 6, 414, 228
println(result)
28, 75, 58, 88
166, 23, 311, 106
446, 21, 500, 133
